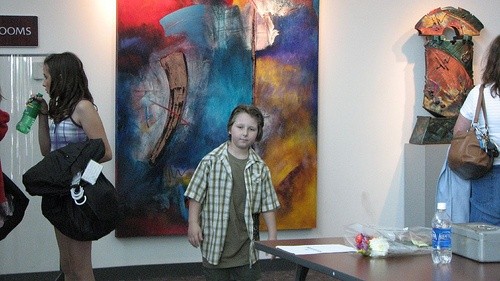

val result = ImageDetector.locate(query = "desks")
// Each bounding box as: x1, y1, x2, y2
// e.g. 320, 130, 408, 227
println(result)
254, 237, 500, 281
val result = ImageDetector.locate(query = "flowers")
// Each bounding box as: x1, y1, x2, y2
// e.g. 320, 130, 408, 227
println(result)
354, 233, 432, 258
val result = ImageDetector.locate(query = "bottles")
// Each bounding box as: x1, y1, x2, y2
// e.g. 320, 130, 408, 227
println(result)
16, 93, 43, 134
431, 202, 453, 264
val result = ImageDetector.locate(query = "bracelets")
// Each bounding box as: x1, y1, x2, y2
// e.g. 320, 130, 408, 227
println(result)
39, 110, 49, 116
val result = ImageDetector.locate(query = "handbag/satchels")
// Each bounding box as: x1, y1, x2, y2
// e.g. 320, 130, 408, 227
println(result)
447, 84, 500, 180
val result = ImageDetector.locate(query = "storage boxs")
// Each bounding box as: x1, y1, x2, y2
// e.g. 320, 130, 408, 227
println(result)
452, 223, 500, 262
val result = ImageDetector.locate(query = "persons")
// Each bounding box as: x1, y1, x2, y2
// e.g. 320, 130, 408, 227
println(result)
452, 33, 500, 226
183, 104, 281, 281
0, 87, 10, 210
26, 51, 112, 281
